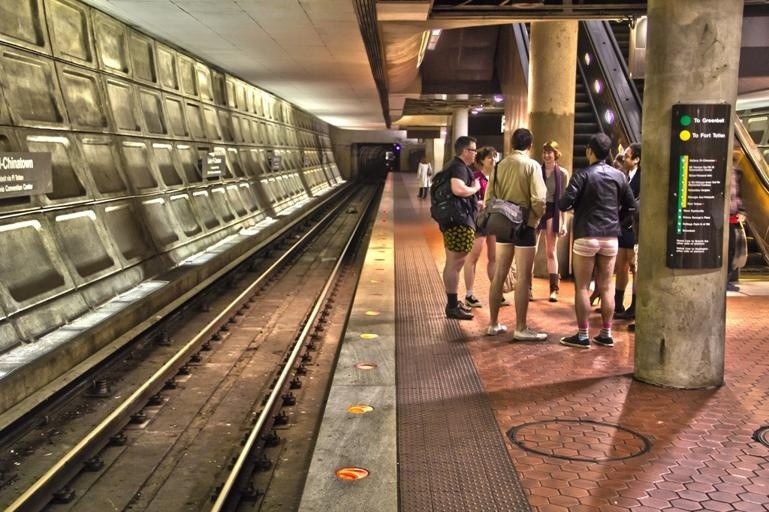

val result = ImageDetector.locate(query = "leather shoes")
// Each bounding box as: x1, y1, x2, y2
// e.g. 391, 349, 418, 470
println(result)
595, 301, 635, 319
445, 301, 474, 320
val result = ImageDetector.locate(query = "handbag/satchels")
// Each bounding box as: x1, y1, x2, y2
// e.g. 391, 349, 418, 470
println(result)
429, 171, 469, 226
474, 197, 528, 242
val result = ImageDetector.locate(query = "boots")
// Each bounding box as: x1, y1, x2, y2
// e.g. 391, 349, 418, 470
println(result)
550, 273, 561, 302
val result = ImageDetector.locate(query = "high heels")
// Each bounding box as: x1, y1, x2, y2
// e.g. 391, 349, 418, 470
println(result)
591, 291, 602, 306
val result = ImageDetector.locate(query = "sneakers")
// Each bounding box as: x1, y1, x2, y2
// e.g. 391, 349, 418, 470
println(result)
487, 323, 507, 336
559, 332, 591, 349
465, 295, 481, 307
514, 327, 547, 341
592, 335, 614, 347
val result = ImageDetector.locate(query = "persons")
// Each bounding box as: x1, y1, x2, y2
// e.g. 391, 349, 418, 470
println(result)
559, 133, 639, 348
417, 157, 432, 199
727, 148, 747, 291
483, 129, 547, 339
443, 136, 508, 319
528, 141, 568, 301
595, 141, 642, 329
